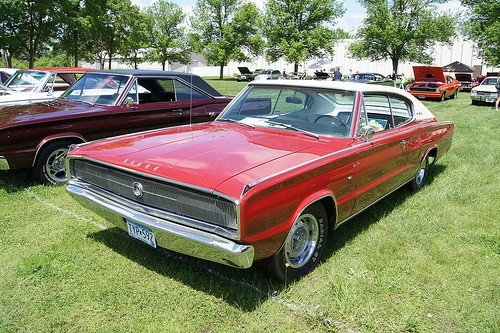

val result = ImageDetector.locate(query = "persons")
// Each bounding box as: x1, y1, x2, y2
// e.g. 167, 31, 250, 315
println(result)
283, 69, 285, 77
400, 74, 405, 89
348, 69, 359, 81
302, 70, 306, 80
496, 71, 500, 111
334, 67, 342, 81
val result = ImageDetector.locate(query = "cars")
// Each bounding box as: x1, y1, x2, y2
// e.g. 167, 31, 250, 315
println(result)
470, 76, 500, 103
64, 79, 455, 285
234, 67, 402, 86
405, 66, 459, 102
0, 67, 272, 187
